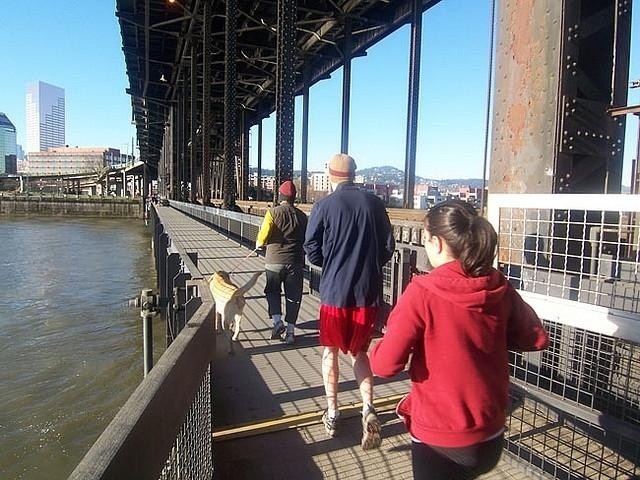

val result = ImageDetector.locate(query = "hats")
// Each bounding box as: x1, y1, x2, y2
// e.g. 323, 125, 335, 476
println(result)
329, 154, 355, 177
279, 180, 296, 196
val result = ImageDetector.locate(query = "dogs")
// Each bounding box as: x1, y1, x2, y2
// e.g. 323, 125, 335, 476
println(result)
208, 271, 263, 341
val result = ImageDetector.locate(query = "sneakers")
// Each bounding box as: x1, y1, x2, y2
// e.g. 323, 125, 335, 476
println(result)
322, 411, 341, 436
361, 410, 382, 449
271, 321, 295, 345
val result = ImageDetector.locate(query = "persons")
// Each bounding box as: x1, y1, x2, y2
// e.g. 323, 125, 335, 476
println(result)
256, 179, 307, 346
303, 154, 395, 450
366, 200, 550, 480
